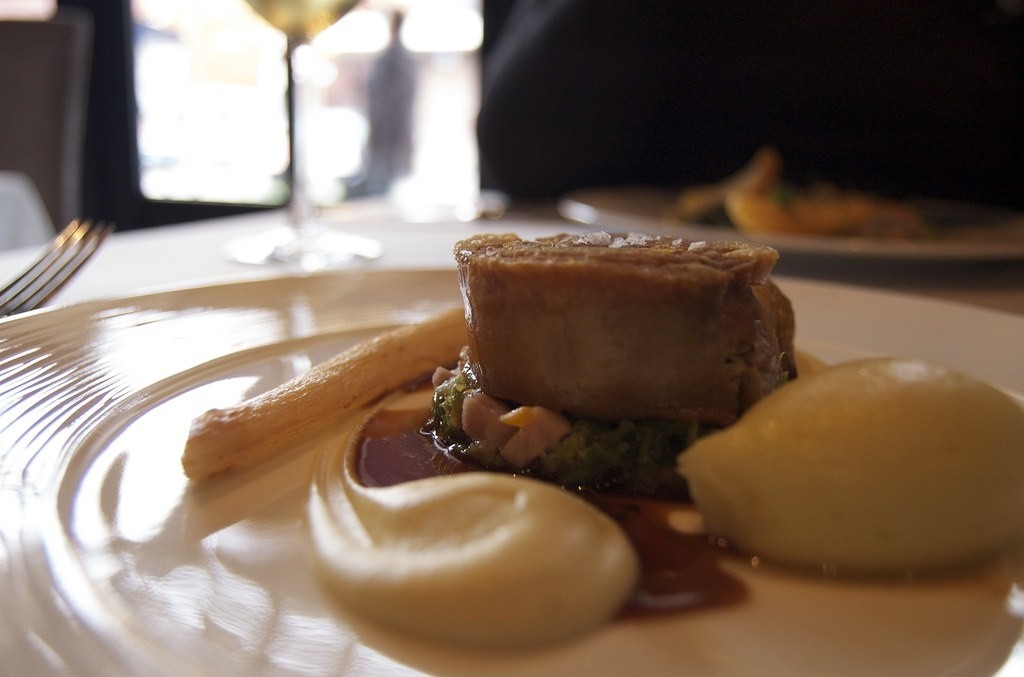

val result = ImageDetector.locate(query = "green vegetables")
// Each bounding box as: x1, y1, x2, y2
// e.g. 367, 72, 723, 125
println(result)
429, 374, 720, 496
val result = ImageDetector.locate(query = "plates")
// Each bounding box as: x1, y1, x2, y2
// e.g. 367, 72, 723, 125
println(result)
0, 268, 1024, 677
557, 191, 1024, 260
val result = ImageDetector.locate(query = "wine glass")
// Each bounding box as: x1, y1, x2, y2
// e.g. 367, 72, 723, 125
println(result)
241, 0, 363, 269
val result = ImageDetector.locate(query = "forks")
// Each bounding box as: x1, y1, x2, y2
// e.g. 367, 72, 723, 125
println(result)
0, 215, 117, 320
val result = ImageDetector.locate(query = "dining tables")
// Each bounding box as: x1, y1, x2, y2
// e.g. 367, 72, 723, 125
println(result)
0, 180, 1024, 414
